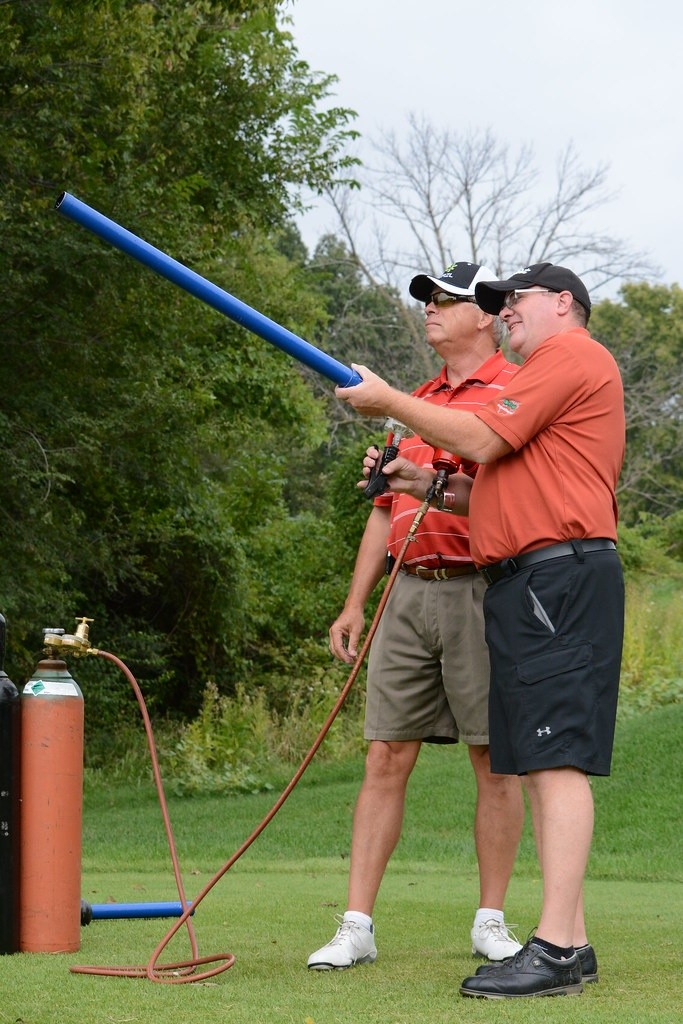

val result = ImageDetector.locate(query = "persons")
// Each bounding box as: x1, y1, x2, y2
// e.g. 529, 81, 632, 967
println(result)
335, 263, 626, 999
308, 260, 522, 972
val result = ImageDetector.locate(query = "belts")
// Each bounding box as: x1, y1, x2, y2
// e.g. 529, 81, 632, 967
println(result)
479, 538, 616, 586
399, 564, 478, 581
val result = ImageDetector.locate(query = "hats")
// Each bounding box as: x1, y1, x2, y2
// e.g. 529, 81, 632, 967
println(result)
409, 262, 500, 302
475, 263, 591, 315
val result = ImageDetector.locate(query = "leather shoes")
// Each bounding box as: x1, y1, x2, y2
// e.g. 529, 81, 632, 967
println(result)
475, 926, 599, 984
459, 941, 583, 1000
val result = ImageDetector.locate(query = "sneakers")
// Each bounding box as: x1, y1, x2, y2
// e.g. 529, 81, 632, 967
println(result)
471, 919, 524, 963
307, 914, 377, 971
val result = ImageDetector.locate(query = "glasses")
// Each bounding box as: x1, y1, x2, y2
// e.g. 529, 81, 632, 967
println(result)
499, 288, 562, 324
426, 291, 477, 308
